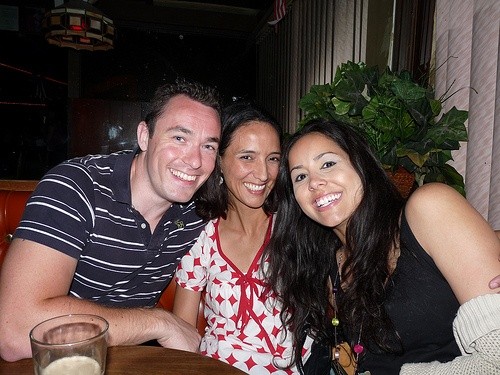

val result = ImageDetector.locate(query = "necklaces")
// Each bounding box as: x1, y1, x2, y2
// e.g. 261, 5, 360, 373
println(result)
332, 246, 363, 375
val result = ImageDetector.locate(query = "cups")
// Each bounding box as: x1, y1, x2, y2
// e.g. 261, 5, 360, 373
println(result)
29, 313, 116, 375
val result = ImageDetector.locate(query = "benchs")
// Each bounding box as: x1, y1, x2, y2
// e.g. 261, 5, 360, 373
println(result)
0, 179, 206, 354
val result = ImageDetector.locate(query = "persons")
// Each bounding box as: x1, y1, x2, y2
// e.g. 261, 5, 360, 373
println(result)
172, 105, 500, 375
0, 78, 224, 362
262, 120, 500, 375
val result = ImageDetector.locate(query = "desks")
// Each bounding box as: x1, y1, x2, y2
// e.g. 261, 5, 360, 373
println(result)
0, 345, 249, 375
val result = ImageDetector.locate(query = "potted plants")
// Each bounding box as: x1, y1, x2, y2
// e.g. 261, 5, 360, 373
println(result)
294, 54, 479, 198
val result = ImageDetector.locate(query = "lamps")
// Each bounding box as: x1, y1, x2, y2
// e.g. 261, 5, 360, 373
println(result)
43, 0, 114, 51
